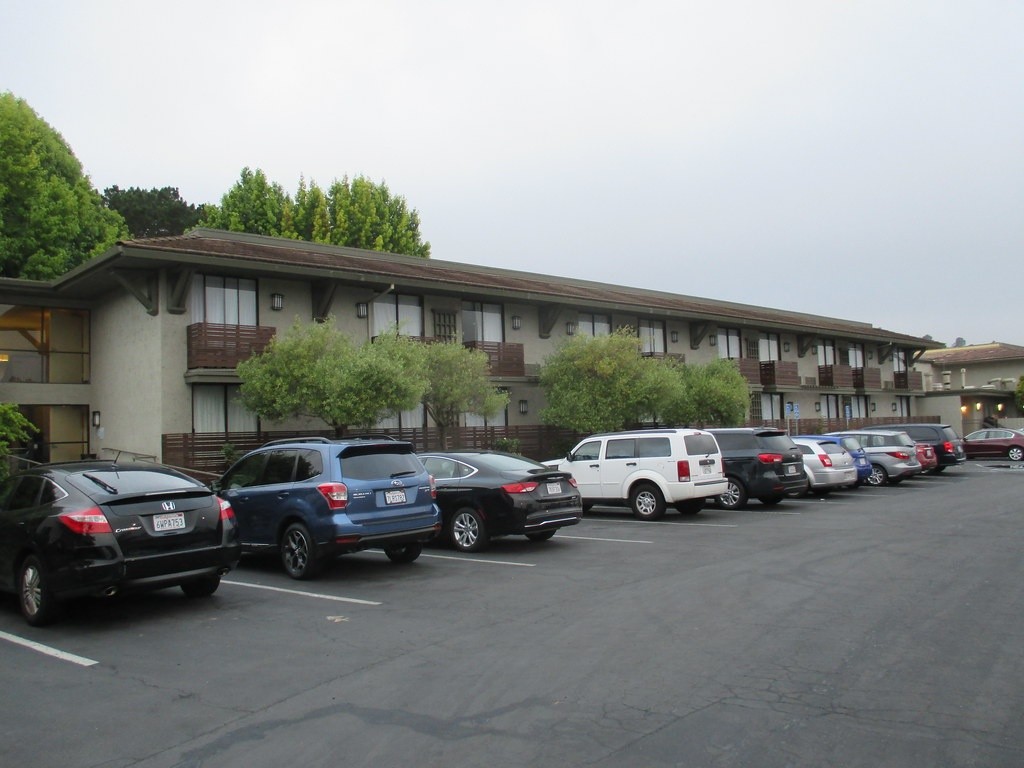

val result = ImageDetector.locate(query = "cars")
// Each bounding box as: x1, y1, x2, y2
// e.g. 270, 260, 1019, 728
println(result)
795, 434, 873, 490
913, 440, 938, 472
960, 429, 1024, 464
0, 460, 241, 628
414, 449, 584, 552
788, 436, 858, 494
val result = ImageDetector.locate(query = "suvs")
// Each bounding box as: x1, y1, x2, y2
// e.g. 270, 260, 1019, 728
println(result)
697, 426, 810, 512
210, 435, 442, 580
536, 427, 730, 522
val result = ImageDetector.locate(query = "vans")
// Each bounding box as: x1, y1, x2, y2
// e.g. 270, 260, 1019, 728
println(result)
859, 423, 967, 473
821, 430, 923, 488
